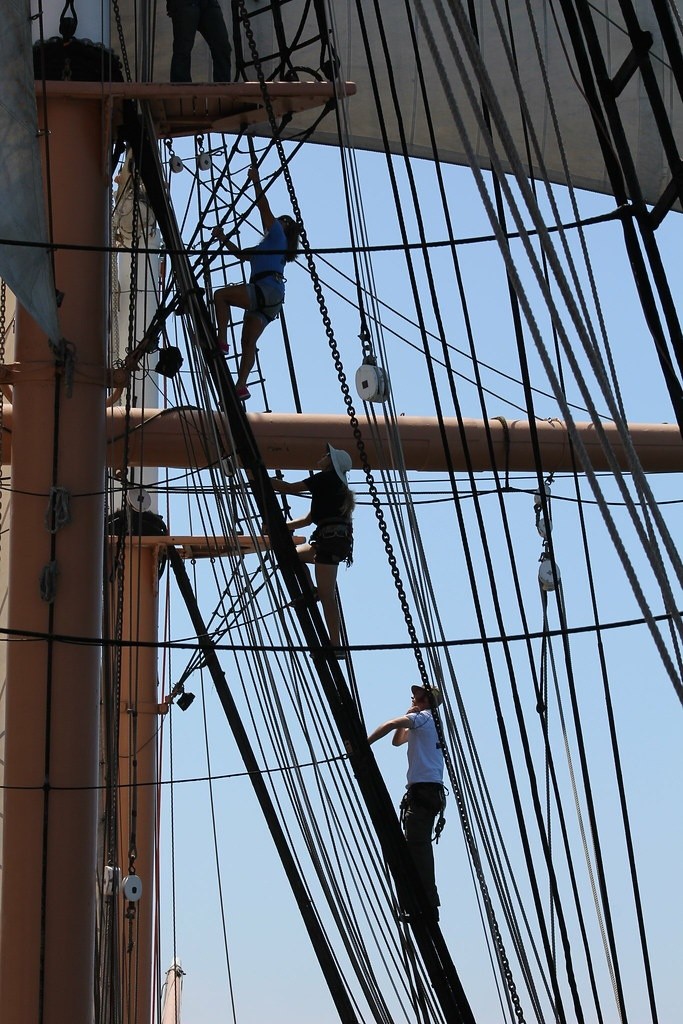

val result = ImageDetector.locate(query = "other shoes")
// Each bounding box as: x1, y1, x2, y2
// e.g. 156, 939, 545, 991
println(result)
288, 587, 321, 606
217, 385, 251, 406
216, 341, 230, 355
396, 908, 439, 922
309, 646, 347, 660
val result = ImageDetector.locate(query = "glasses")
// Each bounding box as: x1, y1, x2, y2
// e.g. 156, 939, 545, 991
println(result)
325, 452, 330, 457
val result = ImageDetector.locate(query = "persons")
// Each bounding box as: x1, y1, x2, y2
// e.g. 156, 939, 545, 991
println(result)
268, 442, 354, 659
166, 0, 232, 83
369, 683, 446, 922
203, 162, 300, 405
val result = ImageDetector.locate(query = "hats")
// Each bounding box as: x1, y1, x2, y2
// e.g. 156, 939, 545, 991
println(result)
411, 683, 444, 707
328, 442, 353, 488
